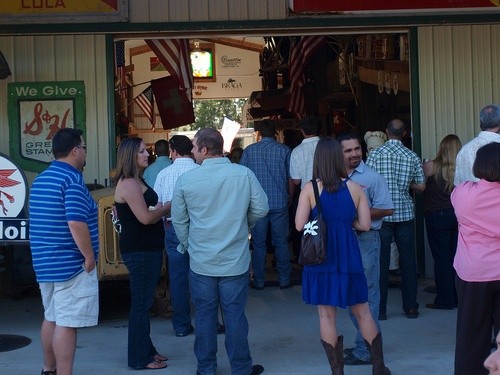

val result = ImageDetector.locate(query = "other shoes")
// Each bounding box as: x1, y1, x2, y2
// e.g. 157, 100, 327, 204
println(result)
251, 283, 264, 291
214, 322, 225, 335
244, 364, 264, 375
378, 313, 387, 321
425, 302, 454, 310
145, 355, 168, 369
405, 308, 419, 319
279, 281, 294, 290
175, 324, 194, 337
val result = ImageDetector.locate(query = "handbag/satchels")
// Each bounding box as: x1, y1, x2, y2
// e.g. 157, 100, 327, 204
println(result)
296, 179, 328, 266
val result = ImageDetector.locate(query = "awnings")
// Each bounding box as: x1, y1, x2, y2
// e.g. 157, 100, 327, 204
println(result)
247, 87, 290, 119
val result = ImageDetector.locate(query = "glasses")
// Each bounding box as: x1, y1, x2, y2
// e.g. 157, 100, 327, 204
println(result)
78, 144, 87, 150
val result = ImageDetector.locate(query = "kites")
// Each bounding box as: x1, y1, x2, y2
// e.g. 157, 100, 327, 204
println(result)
333, 110, 344, 133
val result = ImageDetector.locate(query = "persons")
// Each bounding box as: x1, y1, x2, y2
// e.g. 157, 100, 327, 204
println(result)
170, 127, 269, 375
28, 127, 100, 375
240, 119, 294, 290
423, 134, 463, 312
111, 137, 172, 369
295, 136, 392, 375
289, 114, 321, 191
450, 104, 500, 375
231, 146, 243, 163
141, 134, 225, 336
365, 119, 427, 319
337, 132, 395, 366
363, 131, 387, 158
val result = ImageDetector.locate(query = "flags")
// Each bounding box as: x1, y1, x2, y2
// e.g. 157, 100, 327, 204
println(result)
288, 35, 325, 114
150, 75, 195, 129
134, 85, 154, 127
143, 38, 195, 104
113, 40, 125, 79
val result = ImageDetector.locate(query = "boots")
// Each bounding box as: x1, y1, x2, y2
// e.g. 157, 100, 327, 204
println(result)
319, 335, 345, 375
363, 331, 392, 375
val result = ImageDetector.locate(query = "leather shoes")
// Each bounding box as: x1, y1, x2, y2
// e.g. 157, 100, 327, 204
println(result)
341, 347, 366, 366
